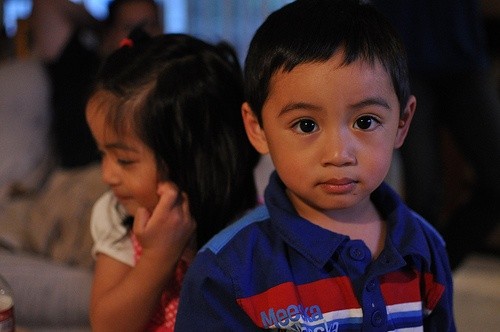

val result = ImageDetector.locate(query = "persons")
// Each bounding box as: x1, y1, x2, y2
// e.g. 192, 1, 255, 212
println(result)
173, 0, 456, 332
85, 18, 277, 332
0, 0, 165, 271
